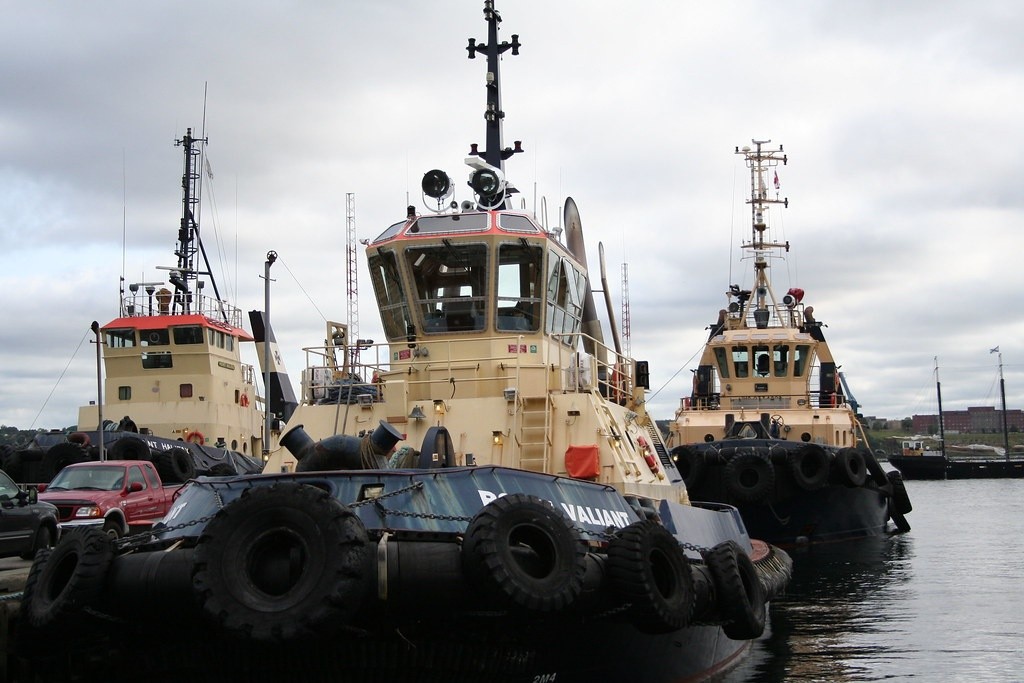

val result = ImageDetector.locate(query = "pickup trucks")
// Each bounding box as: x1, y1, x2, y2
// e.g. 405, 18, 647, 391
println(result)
37, 461, 191, 541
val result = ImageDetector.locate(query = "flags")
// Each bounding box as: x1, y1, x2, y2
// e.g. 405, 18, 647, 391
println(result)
990, 346, 999, 354
206, 157, 214, 179
773, 175, 780, 189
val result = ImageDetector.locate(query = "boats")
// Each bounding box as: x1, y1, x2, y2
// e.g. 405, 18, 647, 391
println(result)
0, 80, 298, 485
663, 139, 912, 550
120, 0, 796, 683
890, 344, 1024, 480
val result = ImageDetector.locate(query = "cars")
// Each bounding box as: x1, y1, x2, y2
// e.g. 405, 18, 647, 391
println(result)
0, 471, 61, 561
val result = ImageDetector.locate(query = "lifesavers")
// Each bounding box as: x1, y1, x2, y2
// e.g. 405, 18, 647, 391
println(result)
886, 469, 915, 514
191, 484, 374, 674
605, 519, 701, 632
831, 392, 836, 408
684, 396, 691, 410
19, 526, 121, 653
669, 442, 871, 504
186, 431, 204, 445
43, 435, 236, 484
706, 540, 769, 640
892, 515, 912, 533
611, 362, 626, 404
459, 492, 589, 624
242, 392, 250, 407
638, 434, 662, 472
64, 432, 91, 449
